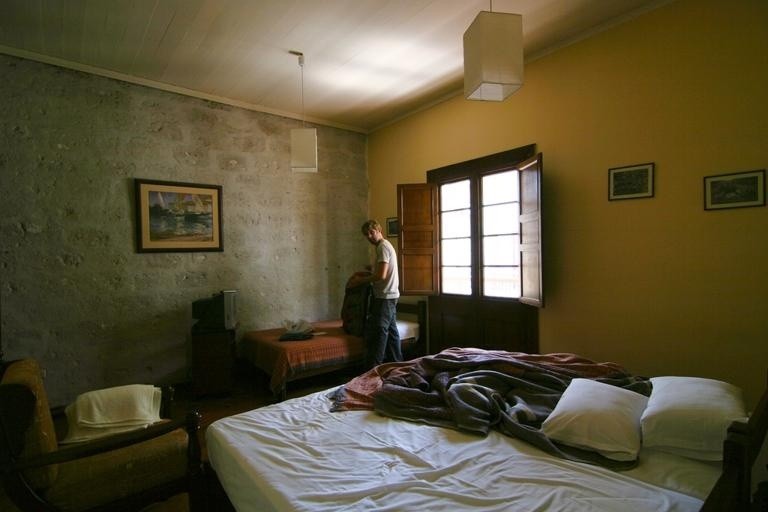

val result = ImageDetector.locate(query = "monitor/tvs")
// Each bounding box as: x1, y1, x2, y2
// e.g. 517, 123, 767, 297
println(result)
192, 290, 239, 330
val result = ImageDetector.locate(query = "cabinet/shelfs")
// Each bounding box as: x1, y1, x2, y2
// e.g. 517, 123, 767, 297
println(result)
182, 328, 238, 399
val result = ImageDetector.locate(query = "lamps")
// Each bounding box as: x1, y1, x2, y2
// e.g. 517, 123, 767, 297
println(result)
462, 0, 524, 103
288, 50, 317, 173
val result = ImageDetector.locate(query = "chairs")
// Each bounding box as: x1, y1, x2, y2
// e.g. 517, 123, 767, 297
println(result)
1, 358, 202, 511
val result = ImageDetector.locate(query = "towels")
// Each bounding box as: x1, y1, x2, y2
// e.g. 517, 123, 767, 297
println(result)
58, 383, 163, 446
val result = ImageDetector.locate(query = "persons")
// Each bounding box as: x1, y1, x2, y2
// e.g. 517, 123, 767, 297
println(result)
360, 219, 405, 366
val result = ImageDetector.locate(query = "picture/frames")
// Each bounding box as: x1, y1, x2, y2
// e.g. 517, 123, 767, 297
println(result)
386, 217, 401, 237
135, 178, 224, 253
608, 162, 765, 211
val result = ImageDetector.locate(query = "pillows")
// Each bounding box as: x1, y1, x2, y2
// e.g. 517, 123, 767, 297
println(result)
539, 376, 750, 462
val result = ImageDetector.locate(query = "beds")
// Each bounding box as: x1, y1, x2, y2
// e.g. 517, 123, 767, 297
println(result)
243, 301, 426, 402
191, 346, 768, 512
